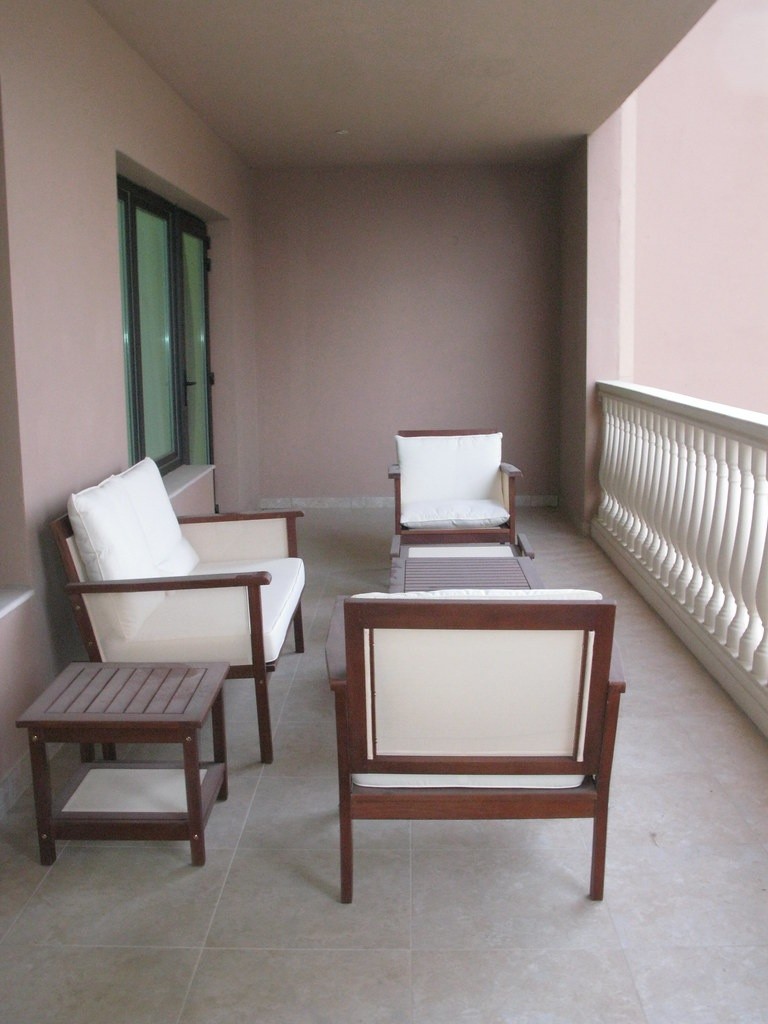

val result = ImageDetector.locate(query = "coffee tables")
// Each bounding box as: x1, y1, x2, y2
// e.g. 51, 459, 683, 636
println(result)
16, 661, 230, 866
390, 557, 545, 592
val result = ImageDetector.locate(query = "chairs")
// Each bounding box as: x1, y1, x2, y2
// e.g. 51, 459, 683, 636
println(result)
326, 590, 626, 903
388, 428, 535, 558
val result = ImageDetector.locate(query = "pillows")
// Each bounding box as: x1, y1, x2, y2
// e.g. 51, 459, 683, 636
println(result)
399, 498, 511, 528
67, 457, 200, 638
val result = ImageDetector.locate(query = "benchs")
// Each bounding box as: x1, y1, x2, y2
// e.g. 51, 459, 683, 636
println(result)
48, 458, 305, 765
